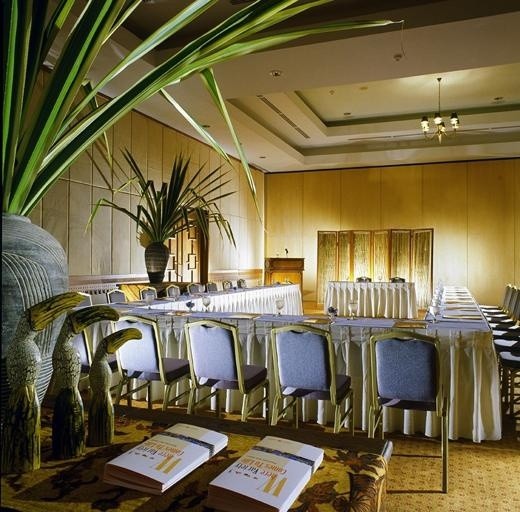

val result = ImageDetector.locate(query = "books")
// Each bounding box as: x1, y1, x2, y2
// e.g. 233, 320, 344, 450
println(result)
103, 423, 228, 496
206, 435, 325, 512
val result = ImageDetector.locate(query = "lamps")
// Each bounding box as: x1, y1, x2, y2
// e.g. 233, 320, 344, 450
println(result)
421, 78, 459, 143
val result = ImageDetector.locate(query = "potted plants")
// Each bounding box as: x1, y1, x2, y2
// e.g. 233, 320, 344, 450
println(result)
0, 0, 404, 437
84, 147, 236, 283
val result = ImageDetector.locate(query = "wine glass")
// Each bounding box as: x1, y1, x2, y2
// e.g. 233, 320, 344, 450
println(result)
349, 302, 358, 320
241, 281, 247, 292
257, 279, 262, 288
224, 282, 230, 294
201, 297, 210, 313
428, 283, 445, 323
275, 299, 285, 318
199, 284, 205, 297
146, 295, 153, 311
171, 287, 178, 301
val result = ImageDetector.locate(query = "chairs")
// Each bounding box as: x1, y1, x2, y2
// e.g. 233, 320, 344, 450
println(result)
368, 332, 448, 493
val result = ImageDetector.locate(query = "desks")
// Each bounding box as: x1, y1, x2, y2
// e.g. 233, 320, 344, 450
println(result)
1, 395, 393, 511
